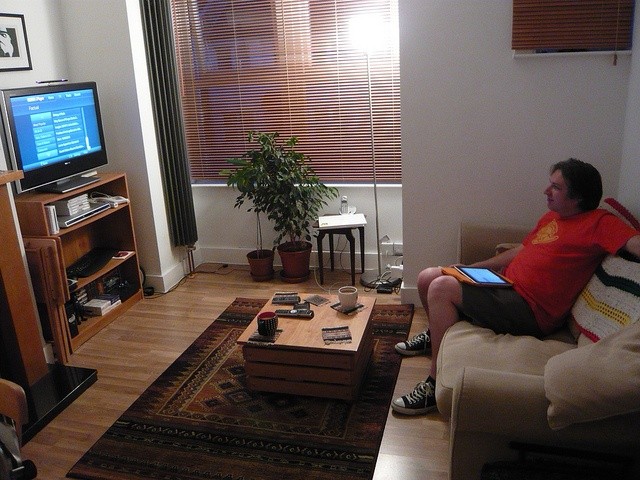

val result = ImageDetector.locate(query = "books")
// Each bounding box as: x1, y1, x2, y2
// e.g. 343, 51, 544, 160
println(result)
70, 288, 123, 317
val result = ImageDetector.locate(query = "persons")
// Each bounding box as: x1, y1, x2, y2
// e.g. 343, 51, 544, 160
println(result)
390, 157, 640, 416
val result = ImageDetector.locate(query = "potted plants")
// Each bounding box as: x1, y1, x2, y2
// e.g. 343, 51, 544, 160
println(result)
226, 128, 339, 284
219, 157, 277, 281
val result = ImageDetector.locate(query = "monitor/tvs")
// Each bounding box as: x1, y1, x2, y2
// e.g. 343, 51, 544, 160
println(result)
0, 82, 109, 195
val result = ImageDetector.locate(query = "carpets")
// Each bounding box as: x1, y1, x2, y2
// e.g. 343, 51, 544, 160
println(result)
65, 296, 416, 480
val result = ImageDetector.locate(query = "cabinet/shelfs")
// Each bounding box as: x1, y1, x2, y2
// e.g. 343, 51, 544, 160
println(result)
13, 173, 144, 355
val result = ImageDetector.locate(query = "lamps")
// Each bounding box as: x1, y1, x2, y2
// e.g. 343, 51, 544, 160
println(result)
346, 13, 403, 288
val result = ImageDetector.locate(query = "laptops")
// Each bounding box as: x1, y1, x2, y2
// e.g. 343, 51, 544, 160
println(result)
318, 213, 368, 231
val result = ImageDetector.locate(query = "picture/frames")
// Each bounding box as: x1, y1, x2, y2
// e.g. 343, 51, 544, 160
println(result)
0, 13, 31, 72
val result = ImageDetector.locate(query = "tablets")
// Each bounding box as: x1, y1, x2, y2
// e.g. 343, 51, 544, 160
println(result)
454, 266, 511, 285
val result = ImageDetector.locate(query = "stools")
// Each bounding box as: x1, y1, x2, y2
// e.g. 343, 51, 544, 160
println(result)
312, 212, 367, 288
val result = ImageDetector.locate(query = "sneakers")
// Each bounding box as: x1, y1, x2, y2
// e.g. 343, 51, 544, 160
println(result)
394, 330, 430, 357
392, 376, 438, 416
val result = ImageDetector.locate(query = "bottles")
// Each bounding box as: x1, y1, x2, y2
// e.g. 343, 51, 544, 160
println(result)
341, 196, 348, 214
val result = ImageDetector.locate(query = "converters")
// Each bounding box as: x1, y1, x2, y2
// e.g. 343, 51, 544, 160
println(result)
390, 265, 402, 278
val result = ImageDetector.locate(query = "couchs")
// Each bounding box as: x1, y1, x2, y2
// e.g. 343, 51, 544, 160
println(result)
436, 196, 638, 480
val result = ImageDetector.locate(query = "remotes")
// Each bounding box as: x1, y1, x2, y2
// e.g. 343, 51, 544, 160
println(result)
272, 296, 300, 304
275, 309, 315, 318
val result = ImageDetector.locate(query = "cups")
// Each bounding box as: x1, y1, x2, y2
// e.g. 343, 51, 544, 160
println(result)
338, 286, 358, 311
257, 312, 278, 336
